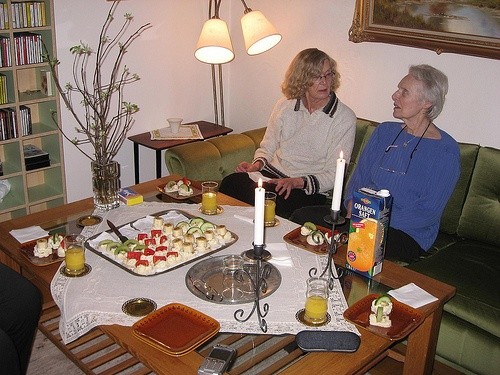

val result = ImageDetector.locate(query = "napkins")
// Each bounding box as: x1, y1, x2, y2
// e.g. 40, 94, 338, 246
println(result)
386, 281, 440, 309
9, 226, 49, 244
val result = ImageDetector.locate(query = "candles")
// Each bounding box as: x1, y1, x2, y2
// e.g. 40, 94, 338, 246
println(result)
331, 151, 345, 210
254, 178, 265, 246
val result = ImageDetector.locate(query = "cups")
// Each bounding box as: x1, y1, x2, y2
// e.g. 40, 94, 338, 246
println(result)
303, 276, 329, 324
64, 234, 85, 272
264, 191, 276, 226
167, 118, 183, 133
201, 180, 219, 214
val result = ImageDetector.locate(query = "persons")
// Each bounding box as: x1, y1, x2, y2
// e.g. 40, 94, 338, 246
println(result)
221, 48, 357, 218
295, 64, 462, 261
0, 262, 43, 375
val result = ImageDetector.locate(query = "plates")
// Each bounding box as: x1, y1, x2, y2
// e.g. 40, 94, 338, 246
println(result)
342, 293, 425, 341
16, 231, 77, 266
185, 256, 281, 304
130, 302, 221, 358
283, 223, 344, 255
156, 178, 201, 200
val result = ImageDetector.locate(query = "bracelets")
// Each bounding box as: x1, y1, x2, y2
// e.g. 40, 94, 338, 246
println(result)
301, 177, 308, 190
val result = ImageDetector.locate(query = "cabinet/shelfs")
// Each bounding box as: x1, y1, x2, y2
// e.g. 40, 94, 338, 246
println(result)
0, 0, 67, 223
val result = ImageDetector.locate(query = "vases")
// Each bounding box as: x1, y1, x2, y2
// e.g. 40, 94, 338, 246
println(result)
90, 158, 120, 218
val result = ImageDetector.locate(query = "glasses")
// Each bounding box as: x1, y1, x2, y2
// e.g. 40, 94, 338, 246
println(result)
312, 70, 335, 82
377, 144, 418, 176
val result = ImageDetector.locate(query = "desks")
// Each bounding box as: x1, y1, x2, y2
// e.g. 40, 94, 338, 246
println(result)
127, 121, 233, 186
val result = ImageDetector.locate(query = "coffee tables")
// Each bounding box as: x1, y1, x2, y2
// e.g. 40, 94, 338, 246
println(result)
0, 174, 456, 375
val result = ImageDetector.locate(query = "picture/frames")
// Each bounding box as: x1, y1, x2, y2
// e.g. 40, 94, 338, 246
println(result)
347, 0, 500, 60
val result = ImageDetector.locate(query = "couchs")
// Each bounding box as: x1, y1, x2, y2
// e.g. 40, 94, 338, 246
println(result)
167, 114, 500, 375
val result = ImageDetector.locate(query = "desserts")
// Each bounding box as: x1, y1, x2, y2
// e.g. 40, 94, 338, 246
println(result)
98, 216, 232, 272
301, 221, 341, 246
34, 233, 70, 257
369, 295, 393, 328
164, 176, 194, 196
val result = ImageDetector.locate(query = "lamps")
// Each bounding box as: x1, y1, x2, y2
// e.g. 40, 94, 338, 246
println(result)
194, 0, 282, 125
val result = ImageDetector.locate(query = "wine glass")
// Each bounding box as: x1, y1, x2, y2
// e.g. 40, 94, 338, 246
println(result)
241, 251, 257, 292
222, 254, 244, 300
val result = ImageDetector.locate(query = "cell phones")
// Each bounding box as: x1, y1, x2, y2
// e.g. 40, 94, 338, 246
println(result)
198, 344, 236, 375
